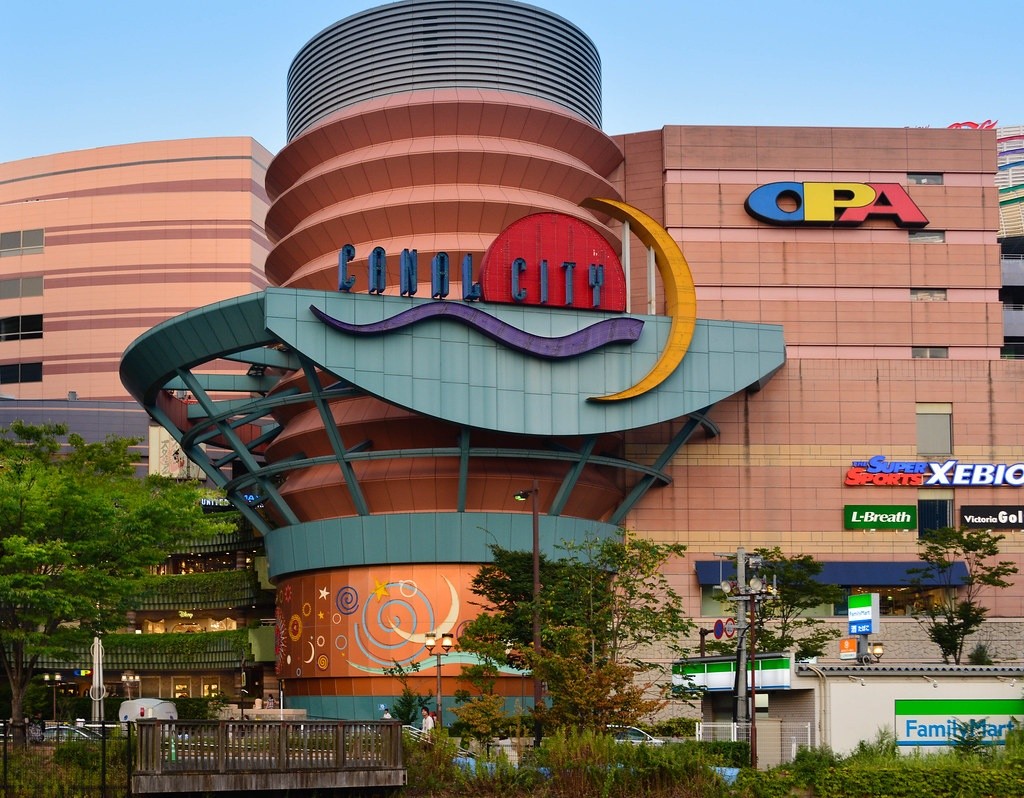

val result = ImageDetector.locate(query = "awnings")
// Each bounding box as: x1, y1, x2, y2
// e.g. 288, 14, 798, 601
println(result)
695, 560, 973, 588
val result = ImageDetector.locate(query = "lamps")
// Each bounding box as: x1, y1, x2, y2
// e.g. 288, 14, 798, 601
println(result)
872, 643, 884, 663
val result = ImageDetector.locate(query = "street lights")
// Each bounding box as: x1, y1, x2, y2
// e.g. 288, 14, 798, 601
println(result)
423, 633, 453, 731
513, 485, 539, 736
722, 578, 765, 772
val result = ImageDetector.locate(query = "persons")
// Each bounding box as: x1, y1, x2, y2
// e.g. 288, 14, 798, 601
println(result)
266, 694, 277, 710
383, 708, 392, 718
420, 706, 435, 736
85, 688, 90, 697
221, 692, 225, 696
27, 709, 46, 744
227, 716, 235, 738
242, 715, 251, 736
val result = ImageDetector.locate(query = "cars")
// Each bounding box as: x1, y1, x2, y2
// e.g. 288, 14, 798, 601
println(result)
45, 724, 102, 743
604, 725, 666, 747
